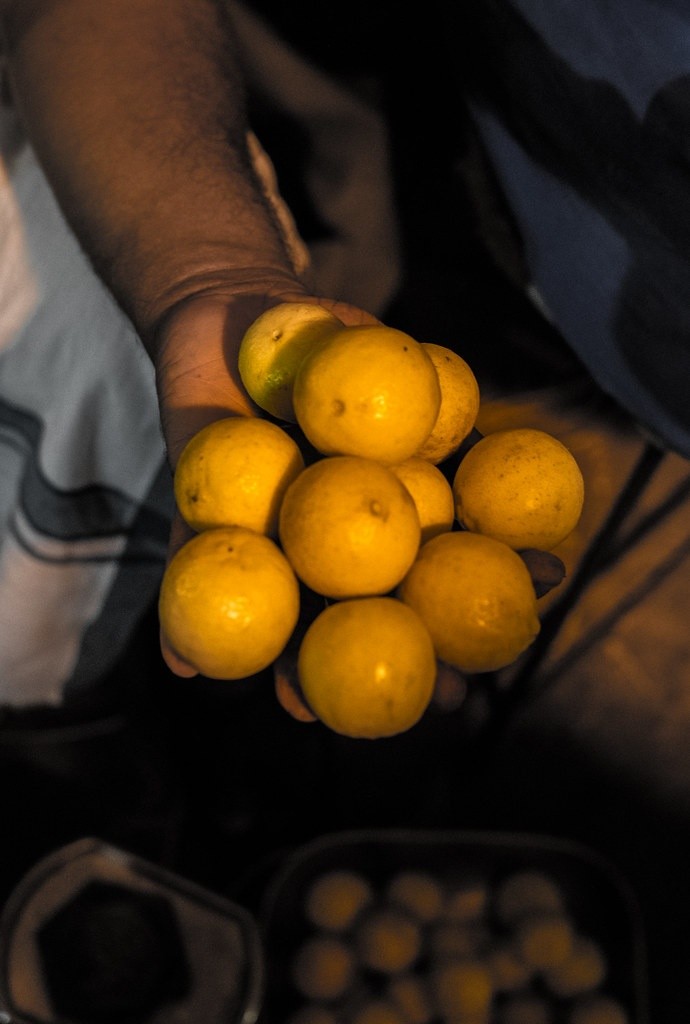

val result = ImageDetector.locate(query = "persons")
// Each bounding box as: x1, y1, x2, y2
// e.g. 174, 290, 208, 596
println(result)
0, 0, 575, 735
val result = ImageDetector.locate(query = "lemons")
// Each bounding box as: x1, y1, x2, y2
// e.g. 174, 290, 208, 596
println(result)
157, 302, 584, 739
